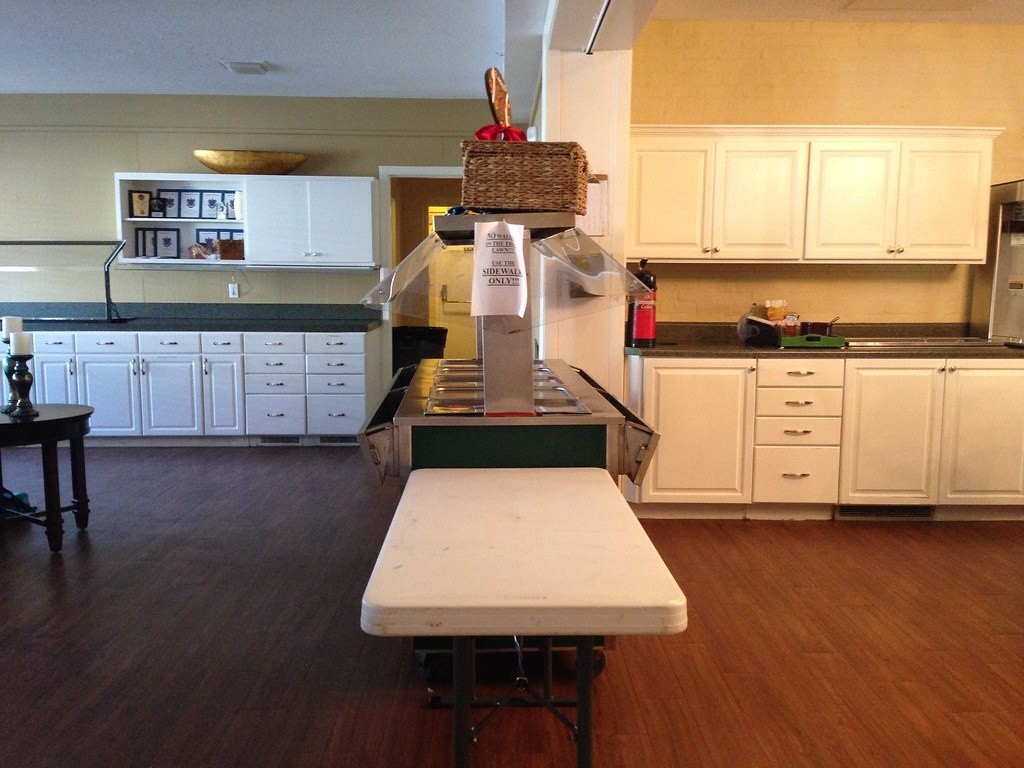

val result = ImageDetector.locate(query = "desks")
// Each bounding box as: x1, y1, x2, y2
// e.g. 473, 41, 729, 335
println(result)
361, 467, 687, 768
0, 403, 94, 552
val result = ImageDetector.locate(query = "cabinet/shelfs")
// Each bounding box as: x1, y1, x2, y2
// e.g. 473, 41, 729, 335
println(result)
33, 331, 77, 448
244, 332, 306, 446
627, 138, 810, 260
640, 357, 756, 520
834, 358, 1024, 521
746, 358, 846, 520
804, 141, 990, 260
114, 172, 380, 267
0, 332, 36, 447
74, 330, 204, 448
201, 331, 249, 446
304, 327, 382, 446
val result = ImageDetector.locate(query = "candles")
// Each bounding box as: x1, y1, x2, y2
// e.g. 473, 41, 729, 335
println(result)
2, 316, 22, 338
10, 332, 33, 355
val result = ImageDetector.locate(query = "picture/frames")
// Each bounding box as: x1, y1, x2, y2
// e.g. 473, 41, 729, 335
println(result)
196, 229, 244, 247
135, 227, 180, 259
128, 189, 235, 220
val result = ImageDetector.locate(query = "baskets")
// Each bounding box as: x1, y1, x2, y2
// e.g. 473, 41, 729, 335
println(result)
460, 139, 587, 215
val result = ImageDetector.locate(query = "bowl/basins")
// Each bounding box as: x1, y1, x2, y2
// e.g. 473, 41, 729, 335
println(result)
216, 240, 244, 260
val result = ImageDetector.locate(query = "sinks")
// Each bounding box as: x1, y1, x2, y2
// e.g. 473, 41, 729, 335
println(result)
845, 333, 1009, 356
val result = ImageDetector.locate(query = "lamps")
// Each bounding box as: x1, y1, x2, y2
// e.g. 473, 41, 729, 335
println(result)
219, 60, 270, 74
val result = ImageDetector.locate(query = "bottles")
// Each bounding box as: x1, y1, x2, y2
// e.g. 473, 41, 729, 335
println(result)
778, 321, 800, 337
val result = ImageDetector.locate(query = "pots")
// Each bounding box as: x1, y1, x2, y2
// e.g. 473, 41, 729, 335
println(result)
800, 317, 839, 342
